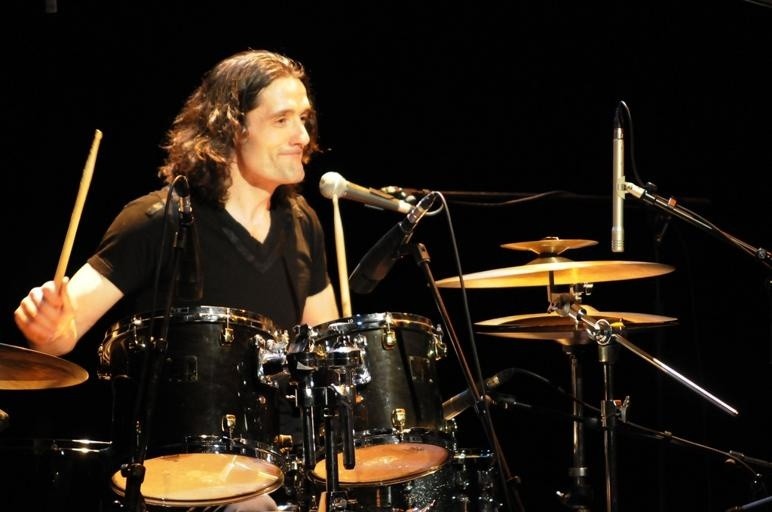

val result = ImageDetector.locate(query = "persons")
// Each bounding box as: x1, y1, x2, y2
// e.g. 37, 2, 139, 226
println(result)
14, 50, 340, 360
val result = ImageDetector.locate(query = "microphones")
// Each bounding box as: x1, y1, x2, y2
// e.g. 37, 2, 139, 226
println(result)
612, 129, 625, 253
441, 366, 516, 422
320, 171, 413, 214
174, 177, 203, 303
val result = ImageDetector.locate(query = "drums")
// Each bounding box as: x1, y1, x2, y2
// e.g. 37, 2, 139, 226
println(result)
98, 305, 288, 508
292, 311, 452, 486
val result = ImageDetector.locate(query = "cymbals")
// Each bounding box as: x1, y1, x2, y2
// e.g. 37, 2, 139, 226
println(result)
500, 236, 599, 256
473, 303, 677, 327
0, 339, 90, 390
473, 321, 679, 349
430, 260, 676, 289
